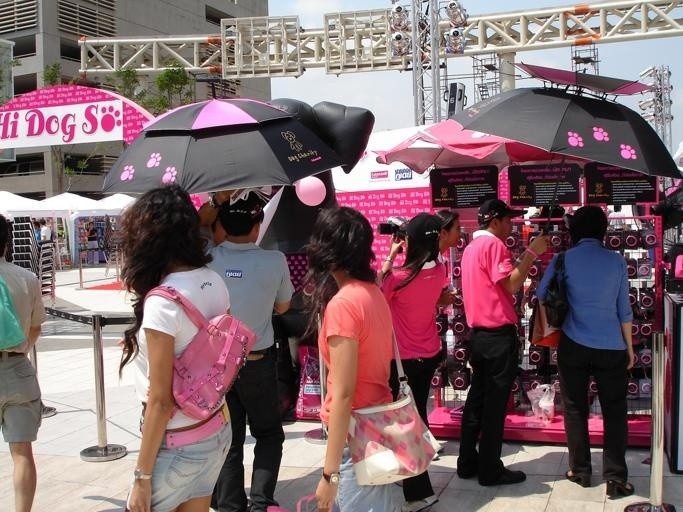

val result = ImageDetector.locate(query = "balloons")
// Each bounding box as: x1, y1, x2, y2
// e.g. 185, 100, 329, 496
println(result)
295, 177, 326, 208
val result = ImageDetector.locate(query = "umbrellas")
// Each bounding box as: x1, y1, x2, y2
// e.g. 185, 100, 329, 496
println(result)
448, 60, 681, 235
375, 118, 592, 176
102, 96, 346, 197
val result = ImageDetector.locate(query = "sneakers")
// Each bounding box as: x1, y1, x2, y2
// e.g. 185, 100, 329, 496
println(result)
401, 494, 439, 512
456, 464, 481, 479
479, 468, 525, 486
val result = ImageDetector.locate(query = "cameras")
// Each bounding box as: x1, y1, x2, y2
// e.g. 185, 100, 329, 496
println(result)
379, 216, 408, 235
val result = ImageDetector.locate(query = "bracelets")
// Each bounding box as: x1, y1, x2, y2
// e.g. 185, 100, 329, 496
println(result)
208, 195, 221, 209
384, 256, 394, 262
526, 249, 539, 259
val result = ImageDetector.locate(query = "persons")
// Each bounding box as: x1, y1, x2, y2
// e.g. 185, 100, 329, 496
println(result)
196, 191, 227, 256
431, 210, 462, 459
536, 205, 637, 497
378, 213, 447, 511
30, 217, 97, 249
306, 206, 408, 511
0, 214, 46, 512
527, 206, 566, 384
207, 200, 293, 511
457, 199, 548, 486
114, 185, 231, 511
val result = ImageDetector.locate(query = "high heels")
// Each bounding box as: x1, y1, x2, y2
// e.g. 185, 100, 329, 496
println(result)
607, 481, 635, 496
566, 469, 592, 488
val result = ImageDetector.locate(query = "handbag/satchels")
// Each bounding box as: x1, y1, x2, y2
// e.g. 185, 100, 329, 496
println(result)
543, 255, 571, 327
145, 287, 256, 421
350, 386, 442, 487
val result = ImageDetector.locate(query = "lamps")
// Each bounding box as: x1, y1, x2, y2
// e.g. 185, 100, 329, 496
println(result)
389, 0, 468, 55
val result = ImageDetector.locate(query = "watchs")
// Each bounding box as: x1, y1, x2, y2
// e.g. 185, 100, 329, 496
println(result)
322, 469, 340, 485
133, 469, 153, 481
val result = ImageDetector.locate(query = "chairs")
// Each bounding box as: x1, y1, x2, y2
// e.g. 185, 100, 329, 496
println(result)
10, 215, 58, 318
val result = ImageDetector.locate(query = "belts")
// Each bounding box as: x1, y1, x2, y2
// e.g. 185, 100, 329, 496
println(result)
0, 352, 25, 358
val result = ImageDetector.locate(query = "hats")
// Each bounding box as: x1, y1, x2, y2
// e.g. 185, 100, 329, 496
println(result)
478, 199, 526, 224
